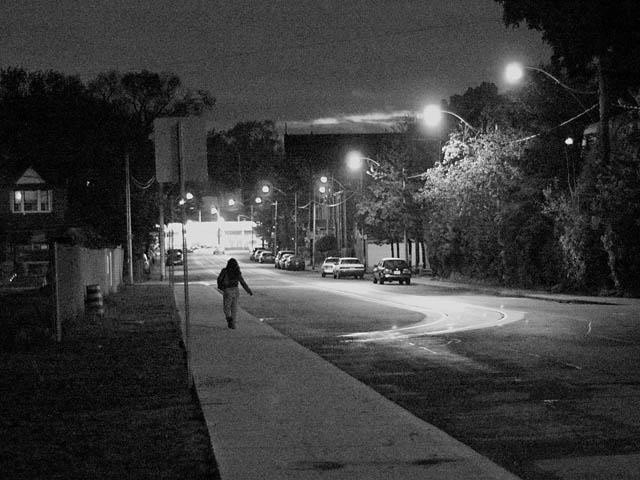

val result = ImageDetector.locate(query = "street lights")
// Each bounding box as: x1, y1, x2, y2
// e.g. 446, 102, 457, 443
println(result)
504, 56, 609, 297
228, 199, 254, 250
211, 208, 229, 249
263, 184, 297, 256
171, 193, 193, 287
347, 151, 408, 267
318, 187, 341, 256
423, 103, 508, 286
255, 196, 277, 257
321, 176, 347, 258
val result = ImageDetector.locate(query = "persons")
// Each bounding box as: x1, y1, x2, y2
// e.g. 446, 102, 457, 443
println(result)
217, 258, 252, 329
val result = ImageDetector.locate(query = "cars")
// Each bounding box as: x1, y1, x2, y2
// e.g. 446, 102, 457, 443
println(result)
187, 241, 225, 254
320, 256, 341, 278
373, 258, 412, 285
167, 249, 182, 265
333, 257, 365, 280
250, 247, 305, 271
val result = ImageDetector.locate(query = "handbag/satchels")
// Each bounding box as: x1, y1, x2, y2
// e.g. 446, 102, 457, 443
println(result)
217, 275, 229, 291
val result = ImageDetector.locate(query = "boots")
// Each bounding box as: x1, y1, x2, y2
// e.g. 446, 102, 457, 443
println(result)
225, 316, 237, 330
